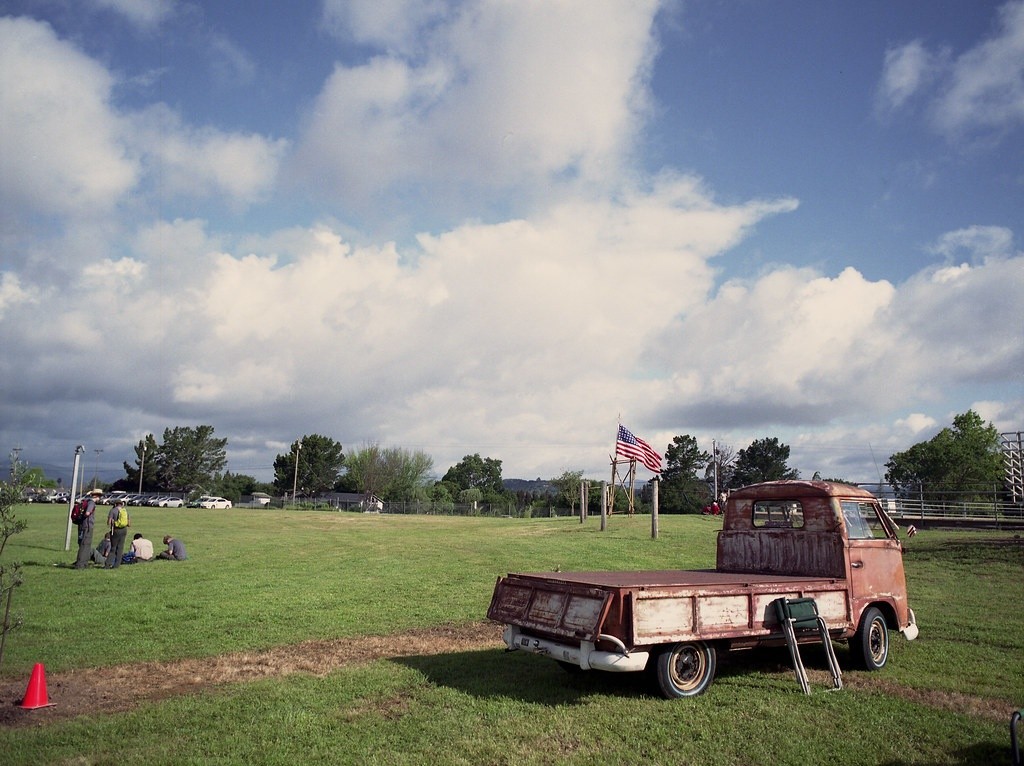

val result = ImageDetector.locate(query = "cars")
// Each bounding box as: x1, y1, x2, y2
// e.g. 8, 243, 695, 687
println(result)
18, 490, 185, 508
186, 495, 211, 508
198, 497, 233, 510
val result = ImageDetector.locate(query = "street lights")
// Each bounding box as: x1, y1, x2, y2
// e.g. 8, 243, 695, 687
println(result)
64, 444, 86, 552
138, 440, 150, 496
291, 440, 304, 508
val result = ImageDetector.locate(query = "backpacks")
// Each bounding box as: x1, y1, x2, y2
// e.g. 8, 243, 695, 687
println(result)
71, 499, 95, 525
113, 507, 128, 528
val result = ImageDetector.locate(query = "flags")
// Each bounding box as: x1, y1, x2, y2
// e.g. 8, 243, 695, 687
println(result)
617, 424, 662, 474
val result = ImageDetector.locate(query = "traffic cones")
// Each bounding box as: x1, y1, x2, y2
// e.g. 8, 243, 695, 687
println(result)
12, 661, 58, 709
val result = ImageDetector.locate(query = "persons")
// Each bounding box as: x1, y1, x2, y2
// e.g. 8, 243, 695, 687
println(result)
74, 489, 105, 569
155, 535, 186, 561
91, 531, 111, 564
702, 489, 728, 515
129, 533, 154, 563
104, 499, 130, 568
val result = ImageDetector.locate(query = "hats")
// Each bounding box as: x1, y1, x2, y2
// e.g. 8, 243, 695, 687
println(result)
90, 489, 104, 495
114, 500, 123, 506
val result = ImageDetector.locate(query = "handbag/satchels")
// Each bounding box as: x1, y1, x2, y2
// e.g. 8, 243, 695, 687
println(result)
121, 552, 137, 564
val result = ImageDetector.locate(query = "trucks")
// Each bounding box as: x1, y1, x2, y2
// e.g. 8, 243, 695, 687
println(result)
484, 478, 919, 697
